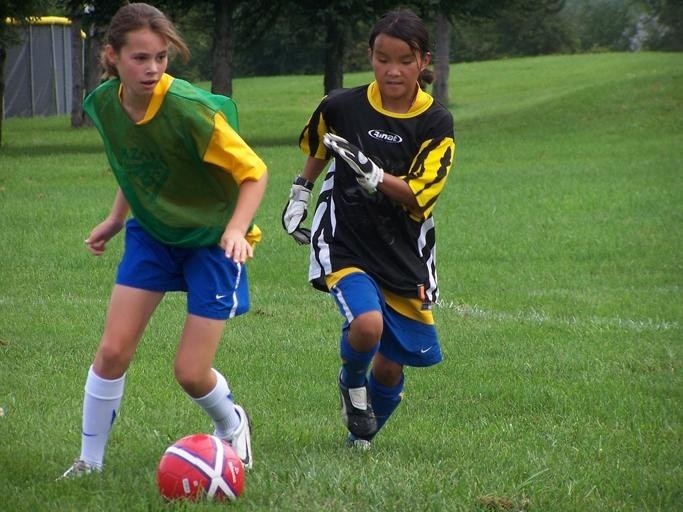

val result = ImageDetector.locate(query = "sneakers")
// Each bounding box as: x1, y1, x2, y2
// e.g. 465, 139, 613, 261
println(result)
212, 406, 254, 472
56, 461, 102, 480
338, 366, 379, 449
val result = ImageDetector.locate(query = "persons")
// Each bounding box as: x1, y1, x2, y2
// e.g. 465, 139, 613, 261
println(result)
53, 1, 270, 483
282, 6, 457, 452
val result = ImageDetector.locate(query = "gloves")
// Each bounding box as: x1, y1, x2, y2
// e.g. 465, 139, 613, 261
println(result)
323, 132, 384, 194
281, 174, 314, 245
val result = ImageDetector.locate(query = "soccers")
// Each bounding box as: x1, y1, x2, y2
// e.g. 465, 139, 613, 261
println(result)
157, 433, 243, 503
